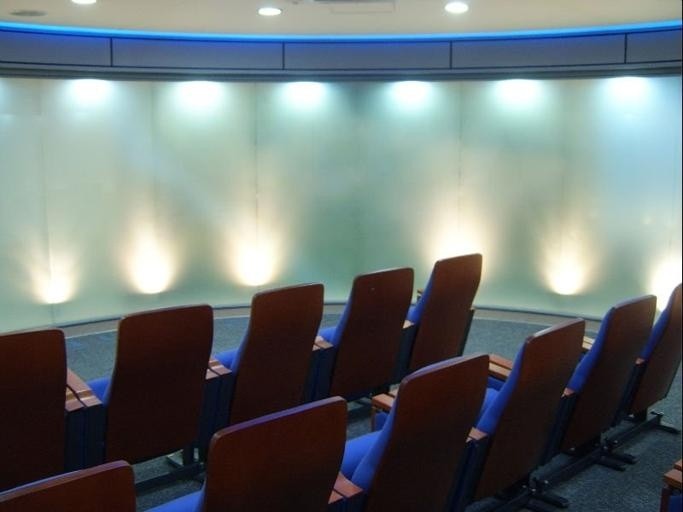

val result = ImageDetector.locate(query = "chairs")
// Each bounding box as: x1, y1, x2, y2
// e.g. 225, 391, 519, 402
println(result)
1, 253, 682, 510
